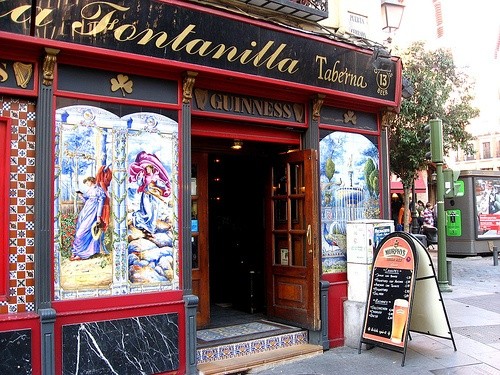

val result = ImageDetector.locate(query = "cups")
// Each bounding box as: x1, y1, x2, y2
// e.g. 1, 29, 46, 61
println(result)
390, 298, 409, 345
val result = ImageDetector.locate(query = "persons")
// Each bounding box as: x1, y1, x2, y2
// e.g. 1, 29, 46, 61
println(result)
398, 200, 437, 232
